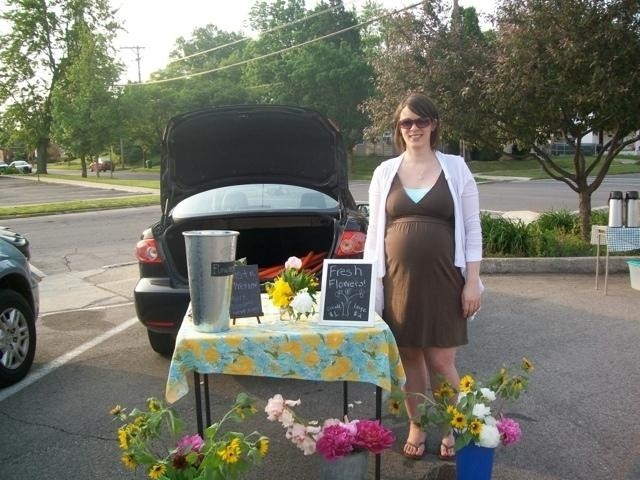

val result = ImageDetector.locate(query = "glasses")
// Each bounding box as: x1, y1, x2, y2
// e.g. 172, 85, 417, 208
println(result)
399, 118, 431, 128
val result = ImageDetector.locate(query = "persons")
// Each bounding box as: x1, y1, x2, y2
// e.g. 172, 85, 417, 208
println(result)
363, 93, 484, 460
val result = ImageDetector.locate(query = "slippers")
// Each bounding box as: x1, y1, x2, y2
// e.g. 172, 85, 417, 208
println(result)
403, 440, 426, 460
438, 441, 457, 460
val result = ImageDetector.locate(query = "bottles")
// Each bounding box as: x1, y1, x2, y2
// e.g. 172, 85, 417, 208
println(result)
608, 190, 639, 229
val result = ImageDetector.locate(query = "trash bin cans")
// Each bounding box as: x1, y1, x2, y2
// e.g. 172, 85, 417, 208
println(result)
146, 160, 152, 169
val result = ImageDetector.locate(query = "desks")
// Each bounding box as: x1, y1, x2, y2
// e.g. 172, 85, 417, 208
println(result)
591, 224, 640, 295
165, 292, 406, 480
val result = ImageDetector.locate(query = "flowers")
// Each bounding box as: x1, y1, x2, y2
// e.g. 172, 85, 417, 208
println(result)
265, 256, 319, 321
266, 393, 394, 459
390, 357, 533, 452
111, 393, 267, 480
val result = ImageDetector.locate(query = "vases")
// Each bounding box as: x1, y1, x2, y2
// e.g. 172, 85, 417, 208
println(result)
280, 306, 293, 321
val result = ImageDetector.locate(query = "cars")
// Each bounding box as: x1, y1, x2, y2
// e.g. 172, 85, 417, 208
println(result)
0, 161, 9, 169
132, 102, 369, 355
88, 157, 116, 173
0, 223, 47, 387
8, 160, 33, 173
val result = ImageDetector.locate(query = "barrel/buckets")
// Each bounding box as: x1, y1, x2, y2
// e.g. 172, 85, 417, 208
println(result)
182, 230, 241, 334
626, 259, 639, 291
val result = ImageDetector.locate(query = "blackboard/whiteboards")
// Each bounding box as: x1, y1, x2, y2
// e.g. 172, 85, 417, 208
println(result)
229, 264, 264, 318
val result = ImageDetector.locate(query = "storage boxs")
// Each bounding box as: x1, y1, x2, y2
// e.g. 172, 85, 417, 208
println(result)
625, 261, 640, 290
